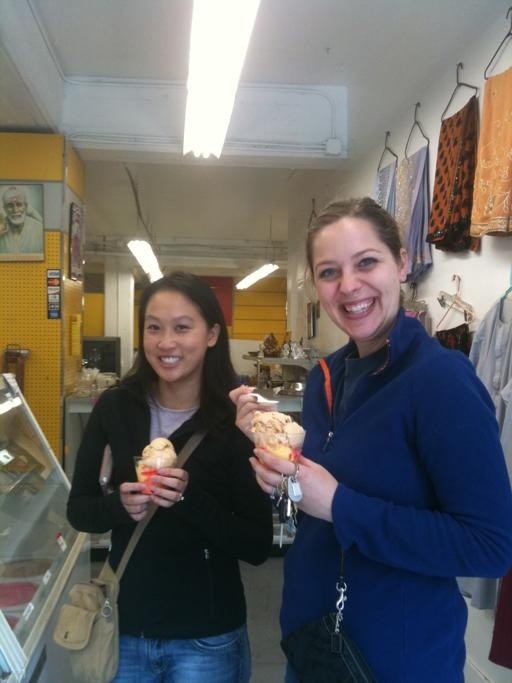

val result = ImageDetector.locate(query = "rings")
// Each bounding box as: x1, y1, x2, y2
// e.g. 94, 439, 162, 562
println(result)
270, 486, 276, 500
176, 492, 182, 502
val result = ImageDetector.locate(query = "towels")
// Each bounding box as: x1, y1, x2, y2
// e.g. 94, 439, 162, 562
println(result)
373, 65, 512, 282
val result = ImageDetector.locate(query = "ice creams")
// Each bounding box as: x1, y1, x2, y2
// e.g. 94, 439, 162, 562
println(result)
134, 438, 178, 495
251, 412, 306, 463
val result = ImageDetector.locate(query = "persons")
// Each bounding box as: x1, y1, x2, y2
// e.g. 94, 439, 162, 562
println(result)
229, 196, 512, 682
67, 272, 274, 683
0, 186, 44, 254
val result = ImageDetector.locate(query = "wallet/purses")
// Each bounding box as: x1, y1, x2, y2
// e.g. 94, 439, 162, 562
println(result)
98, 444, 114, 496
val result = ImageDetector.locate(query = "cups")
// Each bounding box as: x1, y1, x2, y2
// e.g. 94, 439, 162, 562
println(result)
252, 428, 303, 455
133, 455, 175, 490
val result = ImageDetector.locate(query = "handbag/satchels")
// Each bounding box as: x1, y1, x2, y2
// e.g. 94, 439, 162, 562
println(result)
280, 609, 377, 683
53, 558, 119, 683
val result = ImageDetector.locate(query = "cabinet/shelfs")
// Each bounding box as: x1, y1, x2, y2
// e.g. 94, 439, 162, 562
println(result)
241, 346, 320, 414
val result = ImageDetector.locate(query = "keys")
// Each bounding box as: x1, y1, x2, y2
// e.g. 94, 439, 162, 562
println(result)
275, 463, 302, 549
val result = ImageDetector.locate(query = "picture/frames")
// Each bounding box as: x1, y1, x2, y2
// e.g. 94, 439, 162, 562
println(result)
1, 182, 46, 263
68, 200, 84, 280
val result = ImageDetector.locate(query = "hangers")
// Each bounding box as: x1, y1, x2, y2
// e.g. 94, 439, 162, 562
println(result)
484, 6, 511, 80
438, 60, 480, 120
